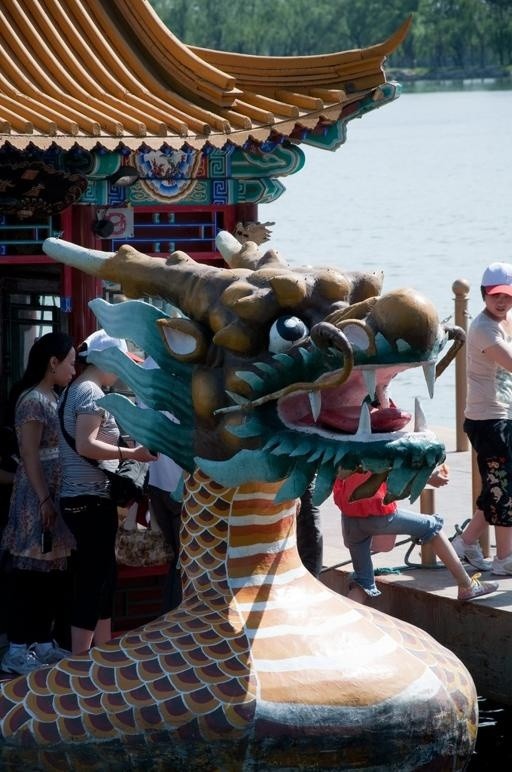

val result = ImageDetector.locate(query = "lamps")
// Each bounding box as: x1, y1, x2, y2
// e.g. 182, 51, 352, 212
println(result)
110, 165, 140, 187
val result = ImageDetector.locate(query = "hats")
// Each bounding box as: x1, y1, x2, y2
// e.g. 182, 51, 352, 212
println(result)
481, 263, 512, 298
77, 329, 144, 363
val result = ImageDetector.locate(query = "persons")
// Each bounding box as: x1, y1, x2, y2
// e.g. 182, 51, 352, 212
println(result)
0, 331, 72, 676
134, 353, 184, 616
295, 473, 325, 581
332, 394, 501, 603
53, 327, 161, 653
449, 262, 512, 576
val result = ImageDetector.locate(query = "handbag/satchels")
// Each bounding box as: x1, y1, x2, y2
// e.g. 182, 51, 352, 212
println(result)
111, 457, 149, 508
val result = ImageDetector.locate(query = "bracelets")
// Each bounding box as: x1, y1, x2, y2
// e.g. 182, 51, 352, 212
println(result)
118, 445, 124, 461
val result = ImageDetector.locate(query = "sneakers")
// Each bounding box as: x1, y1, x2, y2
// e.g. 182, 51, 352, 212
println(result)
352, 572, 375, 589
452, 534, 512, 576
458, 579, 499, 600
0, 639, 72, 676
